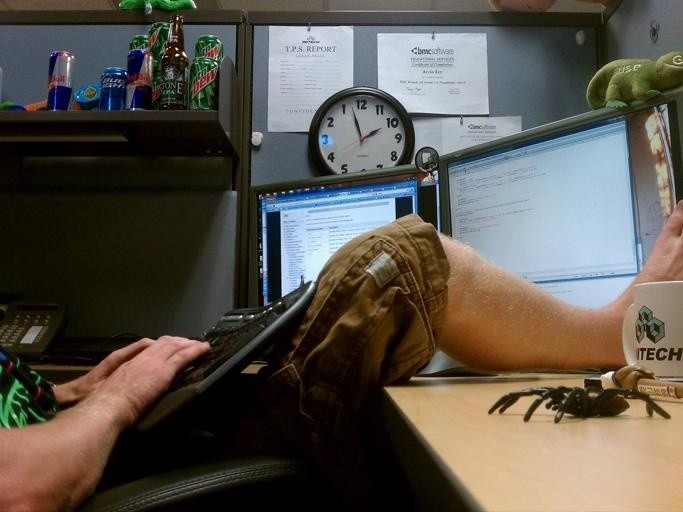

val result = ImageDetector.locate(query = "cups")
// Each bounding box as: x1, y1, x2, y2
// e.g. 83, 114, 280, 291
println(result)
621, 281, 683, 382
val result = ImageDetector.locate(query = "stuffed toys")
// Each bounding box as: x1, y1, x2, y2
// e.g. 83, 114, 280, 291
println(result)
585, 48, 683, 110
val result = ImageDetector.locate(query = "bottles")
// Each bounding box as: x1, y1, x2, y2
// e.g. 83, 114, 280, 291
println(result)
157, 14, 189, 110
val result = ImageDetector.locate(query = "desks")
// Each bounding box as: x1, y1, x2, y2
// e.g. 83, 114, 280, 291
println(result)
23, 358, 683, 511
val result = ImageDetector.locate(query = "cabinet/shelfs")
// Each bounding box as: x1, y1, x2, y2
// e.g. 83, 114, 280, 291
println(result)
0, 6, 248, 383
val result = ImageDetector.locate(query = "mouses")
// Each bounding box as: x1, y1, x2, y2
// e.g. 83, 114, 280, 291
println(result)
417, 350, 503, 379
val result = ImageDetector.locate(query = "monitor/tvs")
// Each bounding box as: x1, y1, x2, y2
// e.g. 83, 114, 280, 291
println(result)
438, 86, 683, 306
248, 164, 440, 311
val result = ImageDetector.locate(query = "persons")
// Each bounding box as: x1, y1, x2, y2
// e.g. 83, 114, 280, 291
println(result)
0, 197, 683, 512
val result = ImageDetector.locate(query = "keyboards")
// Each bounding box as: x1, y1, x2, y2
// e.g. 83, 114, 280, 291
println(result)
136, 279, 318, 428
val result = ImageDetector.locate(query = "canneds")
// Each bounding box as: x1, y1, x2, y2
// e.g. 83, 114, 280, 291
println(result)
46, 50, 74, 112
101, 21, 169, 110
189, 34, 224, 110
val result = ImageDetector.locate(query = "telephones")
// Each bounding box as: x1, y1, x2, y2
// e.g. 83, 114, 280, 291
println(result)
0, 299, 67, 361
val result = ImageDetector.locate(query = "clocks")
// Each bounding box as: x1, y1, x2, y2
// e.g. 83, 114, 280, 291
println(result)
307, 84, 415, 176
